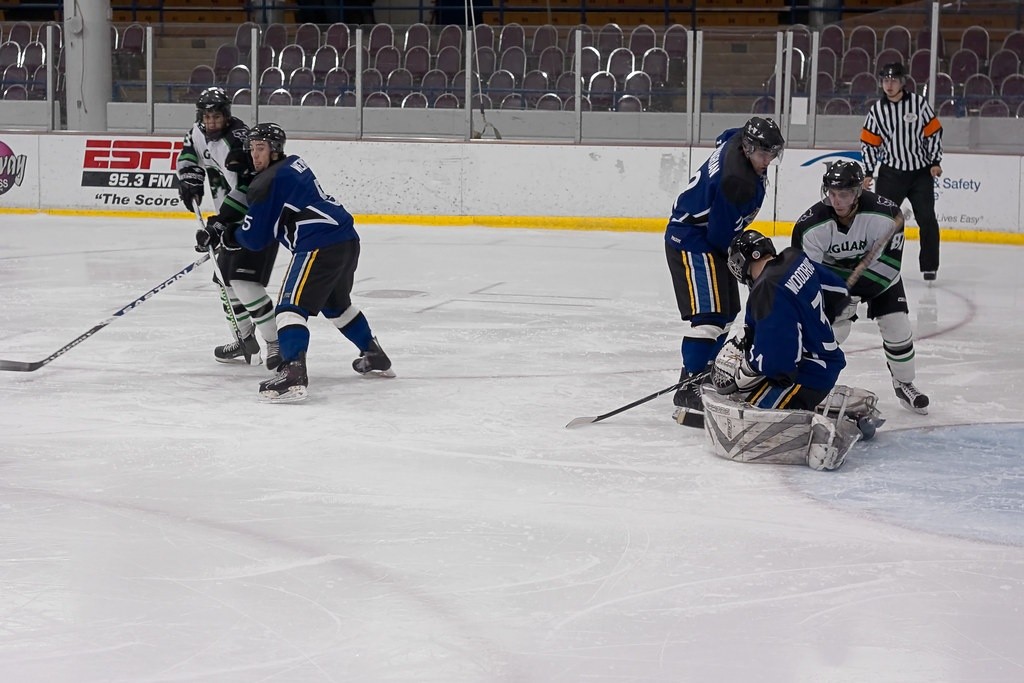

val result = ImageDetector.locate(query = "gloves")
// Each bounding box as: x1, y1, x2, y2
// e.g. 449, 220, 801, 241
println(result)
194, 216, 228, 253
177, 165, 205, 212
224, 149, 258, 176
218, 223, 243, 254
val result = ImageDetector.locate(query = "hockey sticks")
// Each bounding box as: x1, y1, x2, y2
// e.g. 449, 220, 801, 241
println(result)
565, 366, 711, 429
192, 196, 261, 367
0, 246, 222, 372
676, 207, 912, 429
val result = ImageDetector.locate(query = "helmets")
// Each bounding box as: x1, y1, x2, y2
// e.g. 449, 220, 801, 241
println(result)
196, 87, 233, 139
246, 123, 287, 152
822, 160, 864, 188
742, 117, 785, 153
730, 230, 776, 286
880, 62, 906, 77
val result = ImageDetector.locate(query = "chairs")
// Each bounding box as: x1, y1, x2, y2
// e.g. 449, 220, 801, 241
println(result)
178, 21, 688, 112
751, 24, 1024, 119
0, 21, 144, 101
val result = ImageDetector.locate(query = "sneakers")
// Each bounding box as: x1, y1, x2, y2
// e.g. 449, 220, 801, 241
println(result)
923, 270, 936, 287
265, 340, 281, 371
214, 323, 261, 366
672, 367, 704, 428
886, 361, 930, 414
352, 336, 396, 378
257, 350, 309, 402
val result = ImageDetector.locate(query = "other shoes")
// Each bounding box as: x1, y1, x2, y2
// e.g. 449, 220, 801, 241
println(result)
853, 413, 876, 441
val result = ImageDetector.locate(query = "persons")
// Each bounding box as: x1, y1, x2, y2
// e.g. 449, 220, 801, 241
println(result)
220, 123, 396, 404
174, 87, 280, 374
665, 116, 785, 429
859, 62, 944, 283
790, 160, 929, 414
691, 231, 884, 470
